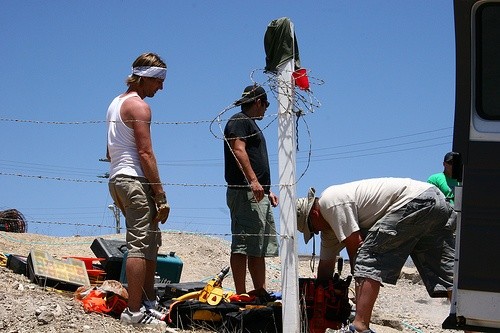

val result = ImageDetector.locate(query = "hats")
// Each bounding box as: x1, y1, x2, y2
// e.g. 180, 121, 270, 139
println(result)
444, 152, 453, 163
235, 86, 266, 106
296, 187, 316, 245
100, 280, 129, 298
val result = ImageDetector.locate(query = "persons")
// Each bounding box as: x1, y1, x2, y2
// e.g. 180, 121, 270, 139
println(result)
107, 52, 170, 325
297, 177, 465, 333
427, 152, 461, 249
224, 85, 279, 294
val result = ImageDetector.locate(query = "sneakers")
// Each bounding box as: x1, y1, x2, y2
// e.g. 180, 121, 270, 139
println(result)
325, 319, 376, 333
120, 300, 169, 326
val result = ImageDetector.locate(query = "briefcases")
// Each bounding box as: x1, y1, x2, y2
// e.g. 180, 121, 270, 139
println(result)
6, 237, 183, 291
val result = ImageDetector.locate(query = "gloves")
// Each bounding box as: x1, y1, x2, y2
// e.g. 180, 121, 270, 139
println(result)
151, 192, 170, 225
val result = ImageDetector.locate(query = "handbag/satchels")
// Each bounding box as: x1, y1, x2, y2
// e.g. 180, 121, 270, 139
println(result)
81, 287, 127, 316
298, 278, 352, 333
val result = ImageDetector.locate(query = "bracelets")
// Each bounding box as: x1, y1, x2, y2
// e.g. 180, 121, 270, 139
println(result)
151, 191, 167, 204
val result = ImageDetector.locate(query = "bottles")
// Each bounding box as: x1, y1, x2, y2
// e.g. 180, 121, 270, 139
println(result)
196, 265, 230, 303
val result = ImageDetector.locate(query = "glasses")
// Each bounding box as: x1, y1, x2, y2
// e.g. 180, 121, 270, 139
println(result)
262, 100, 270, 107
